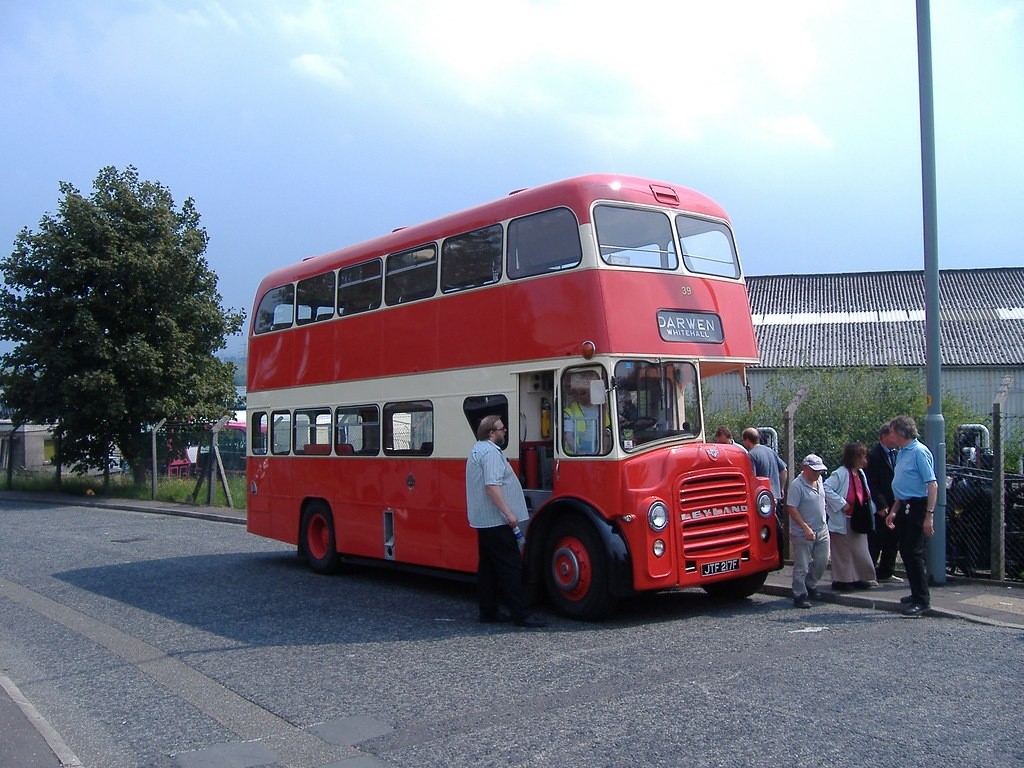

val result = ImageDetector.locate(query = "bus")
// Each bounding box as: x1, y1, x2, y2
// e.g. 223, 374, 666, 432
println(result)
162, 422, 274, 479
244, 171, 788, 621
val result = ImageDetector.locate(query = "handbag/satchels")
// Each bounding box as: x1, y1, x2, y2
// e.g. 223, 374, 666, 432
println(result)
850, 468, 875, 534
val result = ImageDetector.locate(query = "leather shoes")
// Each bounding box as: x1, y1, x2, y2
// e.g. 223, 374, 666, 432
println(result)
479, 612, 513, 623
513, 617, 545, 627
900, 595, 916, 603
902, 602, 931, 615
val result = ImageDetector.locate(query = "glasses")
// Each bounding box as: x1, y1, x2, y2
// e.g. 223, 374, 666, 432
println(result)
495, 428, 506, 432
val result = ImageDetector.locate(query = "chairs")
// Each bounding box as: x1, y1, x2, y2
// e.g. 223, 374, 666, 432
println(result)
269, 273, 490, 332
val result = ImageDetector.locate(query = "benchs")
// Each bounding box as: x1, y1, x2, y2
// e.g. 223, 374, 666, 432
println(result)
303, 444, 355, 457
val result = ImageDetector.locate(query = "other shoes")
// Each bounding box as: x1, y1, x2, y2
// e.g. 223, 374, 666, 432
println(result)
849, 581, 868, 590
832, 581, 854, 592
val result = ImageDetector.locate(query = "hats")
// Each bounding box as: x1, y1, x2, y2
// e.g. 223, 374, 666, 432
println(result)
802, 454, 828, 471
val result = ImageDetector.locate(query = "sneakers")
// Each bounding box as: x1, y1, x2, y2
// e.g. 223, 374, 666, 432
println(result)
878, 575, 904, 583
794, 597, 812, 609
806, 587, 821, 599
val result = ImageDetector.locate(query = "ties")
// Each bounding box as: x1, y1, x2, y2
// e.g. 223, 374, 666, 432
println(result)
889, 451, 895, 470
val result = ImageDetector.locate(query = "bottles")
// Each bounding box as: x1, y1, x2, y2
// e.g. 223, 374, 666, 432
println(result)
526, 446, 538, 489
541, 404, 551, 437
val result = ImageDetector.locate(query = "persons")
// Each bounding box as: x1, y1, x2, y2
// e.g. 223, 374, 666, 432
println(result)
741, 428, 788, 525
562, 378, 611, 455
715, 427, 748, 454
786, 454, 830, 608
822, 442, 876, 591
864, 422, 903, 585
464, 416, 550, 625
885, 415, 938, 615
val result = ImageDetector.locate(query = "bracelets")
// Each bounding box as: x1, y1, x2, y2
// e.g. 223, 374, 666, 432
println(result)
926, 509, 934, 513
